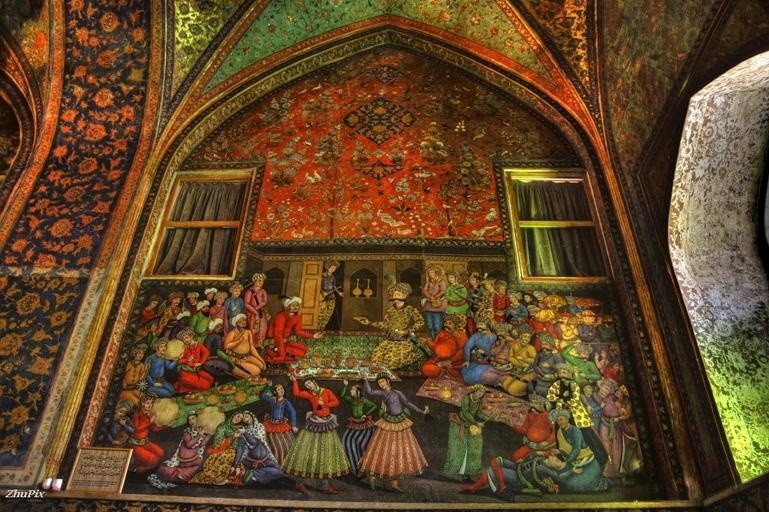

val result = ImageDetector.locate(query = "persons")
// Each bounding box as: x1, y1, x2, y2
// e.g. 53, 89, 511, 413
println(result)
106, 258, 647, 501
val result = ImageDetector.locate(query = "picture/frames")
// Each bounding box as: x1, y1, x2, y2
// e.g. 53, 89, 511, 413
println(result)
65, 444, 134, 493
